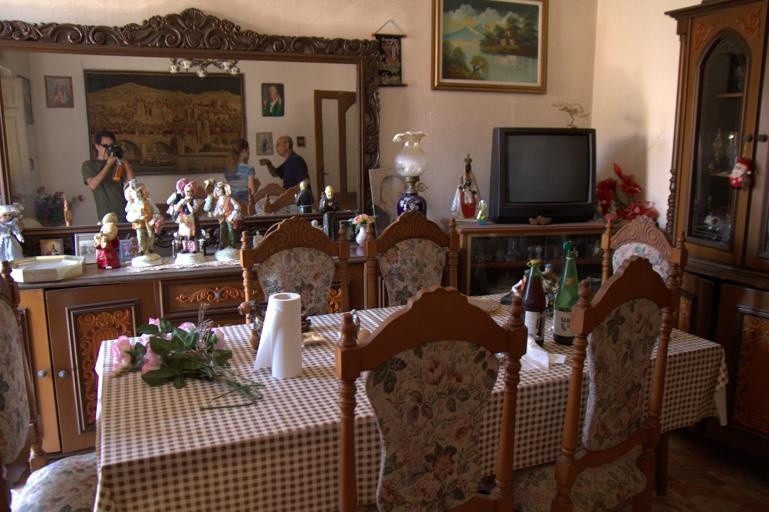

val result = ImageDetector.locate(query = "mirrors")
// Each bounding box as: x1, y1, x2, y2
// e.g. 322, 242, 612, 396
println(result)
0, 7, 380, 255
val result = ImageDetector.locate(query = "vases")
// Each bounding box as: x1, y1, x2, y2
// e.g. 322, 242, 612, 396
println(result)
356, 224, 373, 247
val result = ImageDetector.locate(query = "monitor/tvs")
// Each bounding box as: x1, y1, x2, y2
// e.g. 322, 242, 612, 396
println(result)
488, 127, 598, 224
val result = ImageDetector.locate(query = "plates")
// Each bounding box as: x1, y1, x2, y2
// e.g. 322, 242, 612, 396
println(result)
469, 297, 502, 314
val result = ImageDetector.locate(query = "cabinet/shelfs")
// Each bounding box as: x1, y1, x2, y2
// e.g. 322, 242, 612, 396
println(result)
13, 279, 159, 454
665, 0, 769, 463
159, 263, 366, 328
455, 220, 604, 296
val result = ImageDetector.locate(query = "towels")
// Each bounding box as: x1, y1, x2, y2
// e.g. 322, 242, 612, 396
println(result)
520, 333, 568, 372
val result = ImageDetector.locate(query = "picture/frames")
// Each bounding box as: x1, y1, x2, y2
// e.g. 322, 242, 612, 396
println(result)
256, 132, 274, 155
84, 69, 246, 176
17, 74, 33, 124
431, 0, 549, 94
261, 83, 284, 116
45, 75, 74, 107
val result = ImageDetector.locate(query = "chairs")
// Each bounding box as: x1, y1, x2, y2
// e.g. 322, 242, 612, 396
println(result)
338, 285, 528, 512
0, 260, 97, 511
363, 208, 458, 311
514, 255, 681, 511
601, 216, 688, 497
240, 216, 350, 322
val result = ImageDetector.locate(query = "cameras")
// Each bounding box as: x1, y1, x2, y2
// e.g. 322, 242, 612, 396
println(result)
103, 143, 123, 159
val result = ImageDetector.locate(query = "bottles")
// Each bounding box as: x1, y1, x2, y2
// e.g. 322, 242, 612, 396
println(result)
524, 260, 545, 349
553, 248, 583, 345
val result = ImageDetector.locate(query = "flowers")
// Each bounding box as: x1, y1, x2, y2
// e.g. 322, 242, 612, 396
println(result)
352, 210, 377, 233
109, 319, 265, 407
34, 187, 85, 226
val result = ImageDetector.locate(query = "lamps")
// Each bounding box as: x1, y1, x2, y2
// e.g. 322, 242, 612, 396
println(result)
168, 58, 240, 79
391, 131, 429, 217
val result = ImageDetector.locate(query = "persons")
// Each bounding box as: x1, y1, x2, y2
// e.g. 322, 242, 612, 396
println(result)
0, 299, 31, 512
0, 202, 25, 270
98, 212, 118, 223
123, 178, 244, 254
81, 130, 135, 222
264, 86, 283, 116
258, 136, 313, 205
294, 180, 313, 214
224, 140, 255, 205
319, 186, 340, 241
94, 212, 121, 269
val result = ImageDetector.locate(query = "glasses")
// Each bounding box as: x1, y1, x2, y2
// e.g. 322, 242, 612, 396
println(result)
99, 143, 108, 147
